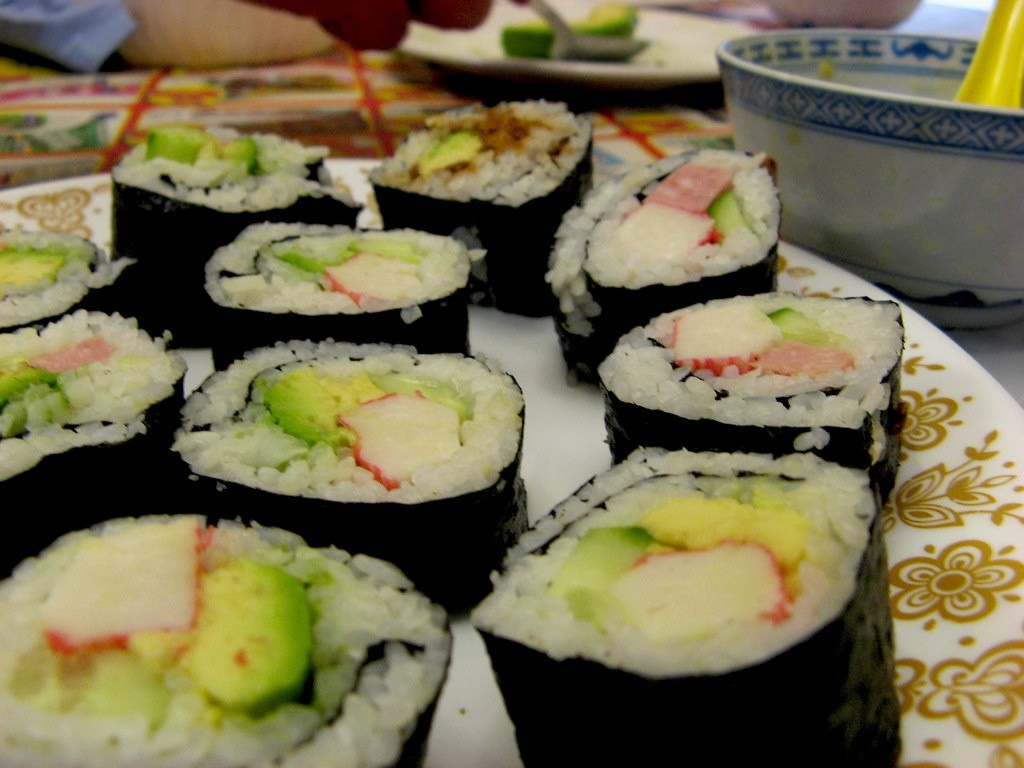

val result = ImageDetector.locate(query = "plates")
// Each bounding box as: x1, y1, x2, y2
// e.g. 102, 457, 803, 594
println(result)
0, 160, 1024, 768
399, 0, 765, 82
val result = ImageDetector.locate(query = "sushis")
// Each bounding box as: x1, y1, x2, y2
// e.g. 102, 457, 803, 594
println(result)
0, 96, 905, 768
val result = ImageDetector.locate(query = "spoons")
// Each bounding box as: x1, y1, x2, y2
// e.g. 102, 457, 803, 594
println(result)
530, 0, 648, 57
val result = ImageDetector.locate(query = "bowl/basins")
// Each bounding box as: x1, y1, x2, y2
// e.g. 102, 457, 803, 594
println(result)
717, 26, 1024, 328
